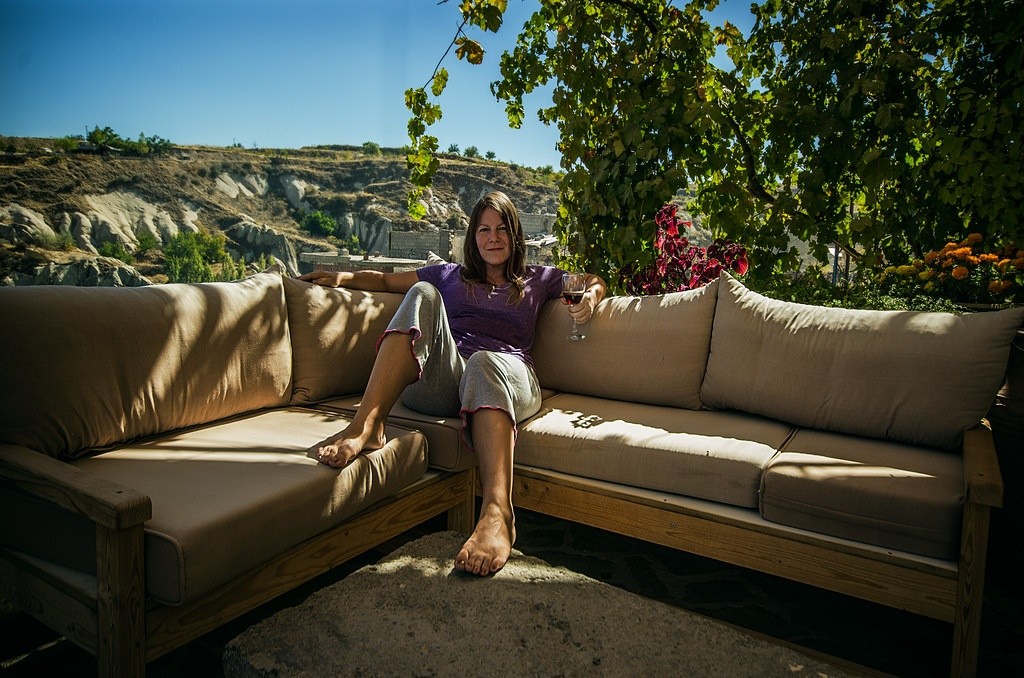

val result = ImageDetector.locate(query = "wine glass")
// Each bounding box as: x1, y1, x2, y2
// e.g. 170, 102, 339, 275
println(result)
561, 273, 587, 342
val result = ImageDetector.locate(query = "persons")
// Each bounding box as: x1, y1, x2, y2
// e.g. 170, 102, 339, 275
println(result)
292, 192, 606, 576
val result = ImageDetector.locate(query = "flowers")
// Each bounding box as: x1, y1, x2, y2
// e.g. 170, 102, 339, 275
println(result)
875, 233, 1024, 305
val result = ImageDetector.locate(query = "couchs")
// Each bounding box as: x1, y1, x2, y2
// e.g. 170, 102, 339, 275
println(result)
0, 272, 1024, 678
